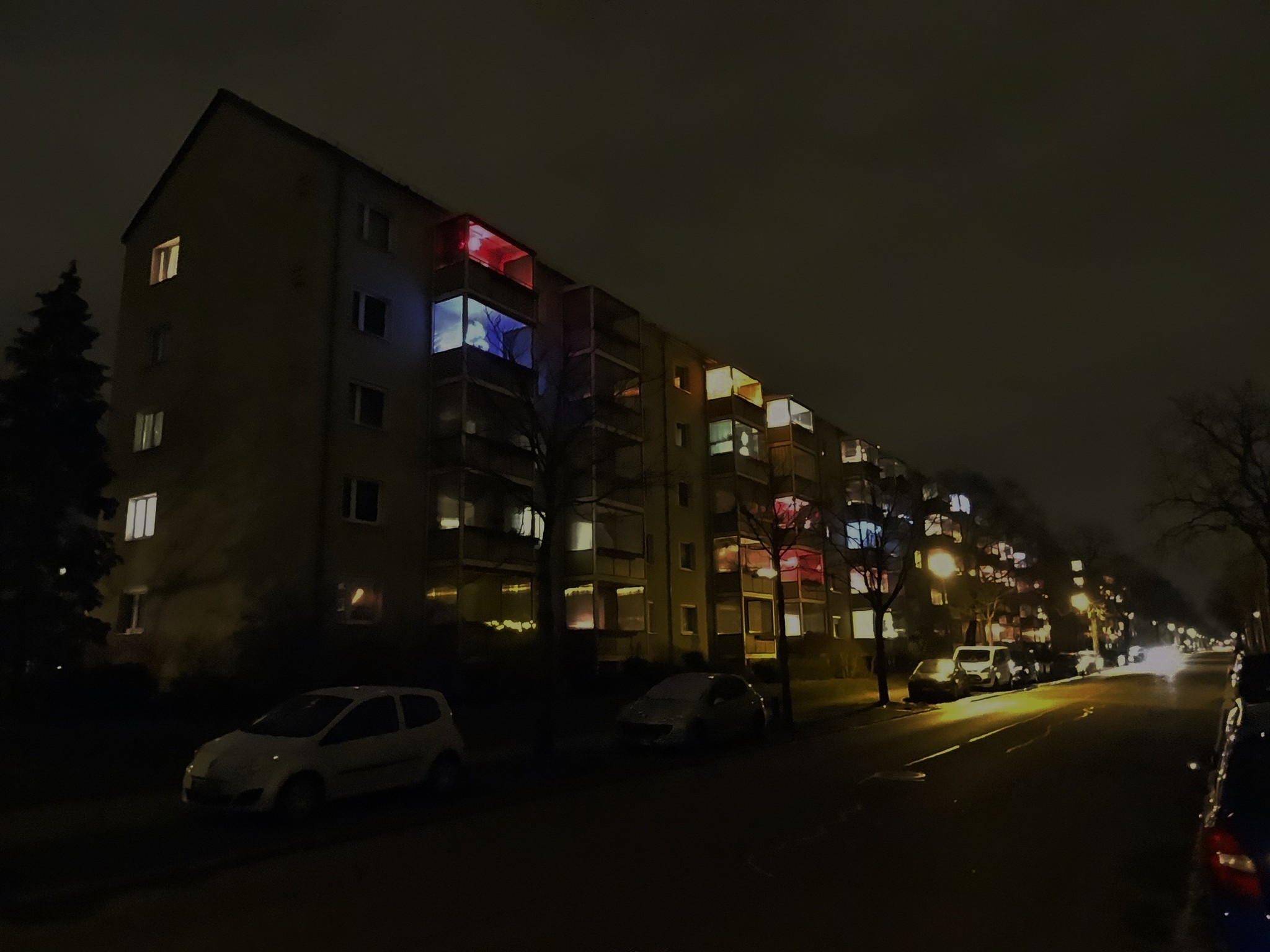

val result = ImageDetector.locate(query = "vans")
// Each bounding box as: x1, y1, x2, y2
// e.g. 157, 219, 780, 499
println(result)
952, 641, 1053, 692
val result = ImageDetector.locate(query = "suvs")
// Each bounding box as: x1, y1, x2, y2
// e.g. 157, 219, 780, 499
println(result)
1202, 696, 1270, 952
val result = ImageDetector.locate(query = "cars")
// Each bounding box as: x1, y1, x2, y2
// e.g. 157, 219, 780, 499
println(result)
616, 671, 768, 752
907, 658, 970, 702
1226, 647, 1270, 701
179, 685, 473, 826
1129, 646, 1147, 663
1052, 650, 1105, 682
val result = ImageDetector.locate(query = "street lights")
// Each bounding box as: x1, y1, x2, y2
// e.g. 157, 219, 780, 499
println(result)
927, 548, 955, 643
